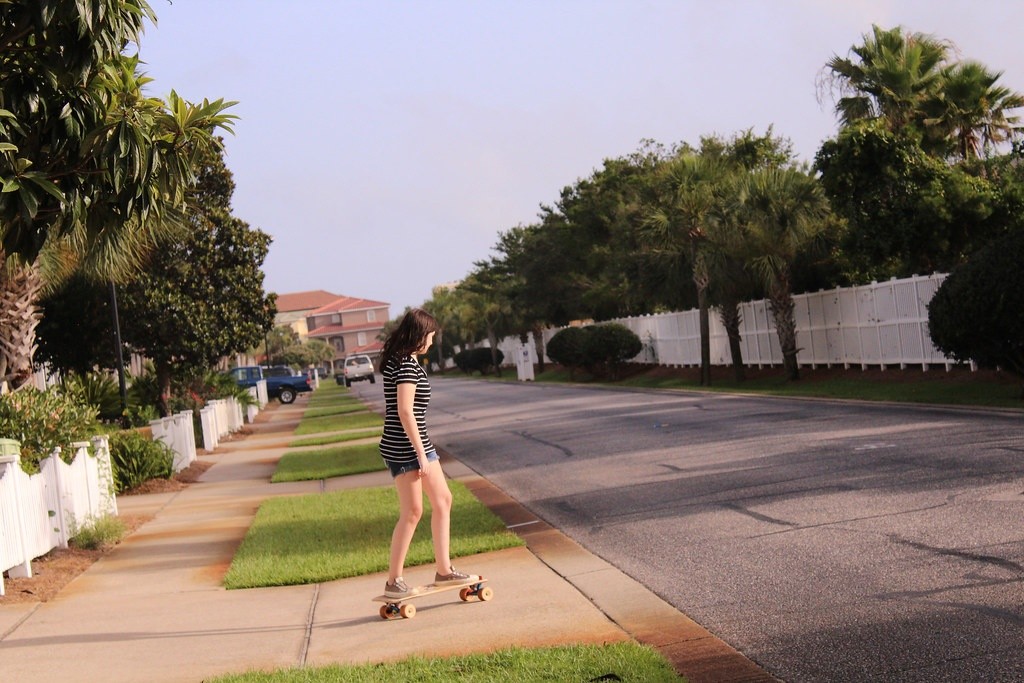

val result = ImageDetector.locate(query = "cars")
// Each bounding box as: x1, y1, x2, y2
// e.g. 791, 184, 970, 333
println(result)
262, 366, 328, 381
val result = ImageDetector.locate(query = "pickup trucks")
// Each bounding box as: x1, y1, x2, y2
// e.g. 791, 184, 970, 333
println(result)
228, 365, 313, 404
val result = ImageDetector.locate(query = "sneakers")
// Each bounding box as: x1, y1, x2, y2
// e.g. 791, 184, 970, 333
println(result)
384, 577, 418, 598
435, 566, 479, 587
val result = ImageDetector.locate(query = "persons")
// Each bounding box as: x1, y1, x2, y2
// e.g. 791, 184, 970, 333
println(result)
379, 308, 479, 598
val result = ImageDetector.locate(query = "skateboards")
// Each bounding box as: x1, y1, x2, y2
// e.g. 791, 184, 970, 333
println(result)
371, 576, 494, 621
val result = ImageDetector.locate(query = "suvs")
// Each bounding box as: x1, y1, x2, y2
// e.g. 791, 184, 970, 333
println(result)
344, 355, 375, 387
